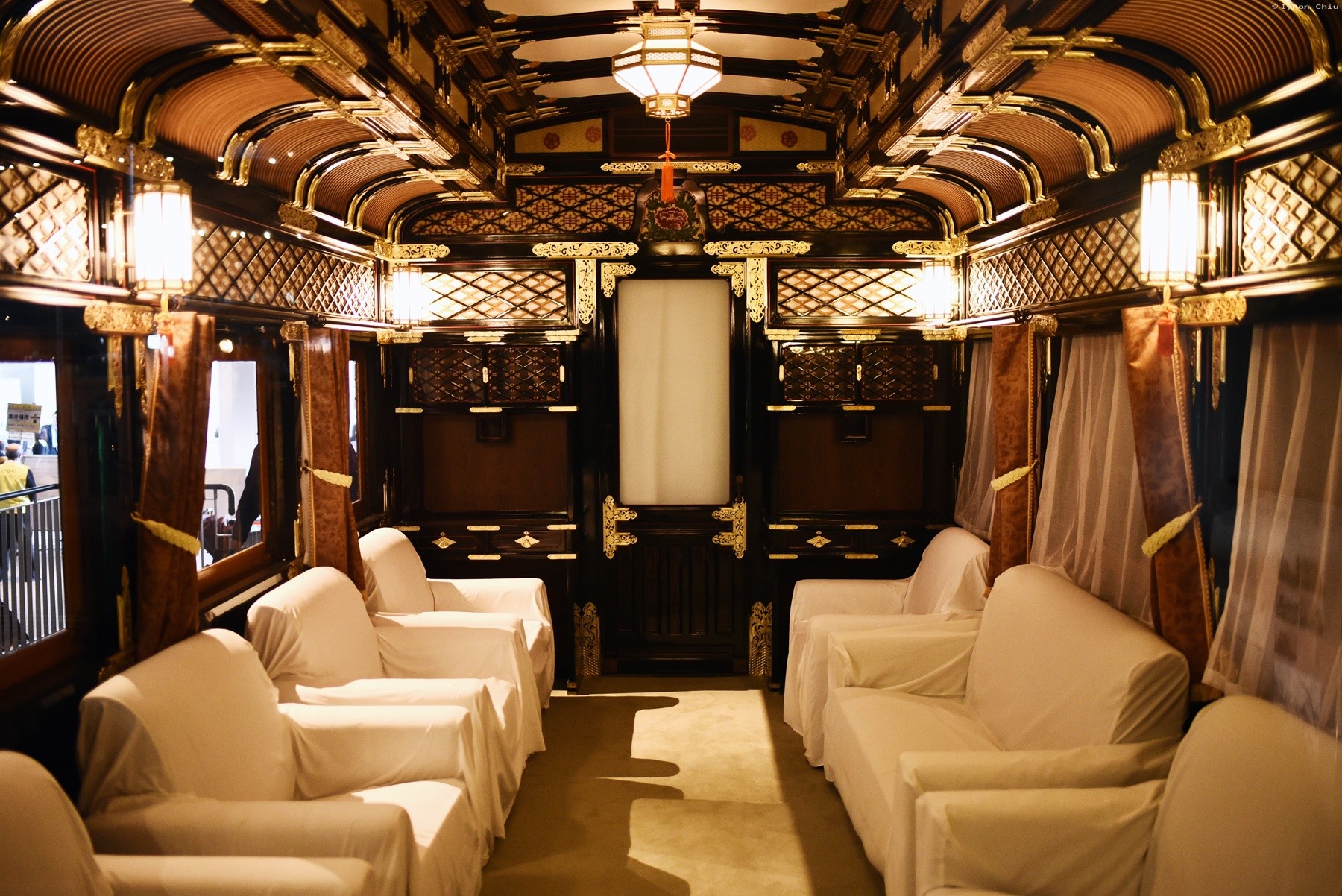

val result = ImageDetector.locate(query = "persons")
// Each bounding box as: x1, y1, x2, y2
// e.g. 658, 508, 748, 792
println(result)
230, 444, 261, 550
0, 442, 40, 583
32, 428, 49, 455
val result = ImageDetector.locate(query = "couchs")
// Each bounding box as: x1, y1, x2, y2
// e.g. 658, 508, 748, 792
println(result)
1, 529, 557, 896
779, 527, 1342, 896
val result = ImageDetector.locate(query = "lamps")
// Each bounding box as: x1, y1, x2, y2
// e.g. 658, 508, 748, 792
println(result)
394, 264, 425, 325
1141, 171, 1226, 287
112, 180, 198, 295
923, 259, 953, 320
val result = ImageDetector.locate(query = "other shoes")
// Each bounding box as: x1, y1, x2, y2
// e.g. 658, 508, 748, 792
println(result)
25, 571, 40, 581
15, 547, 19, 555
0, 574, 4, 582
